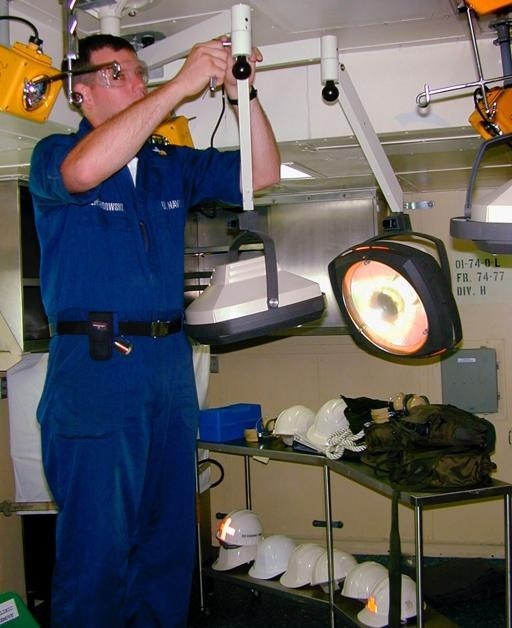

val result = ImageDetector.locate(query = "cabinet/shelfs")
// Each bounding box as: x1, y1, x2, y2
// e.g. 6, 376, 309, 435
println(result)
192, 438, 508, 627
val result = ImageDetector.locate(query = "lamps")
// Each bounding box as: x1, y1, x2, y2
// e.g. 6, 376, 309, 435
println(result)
88, 1, 468, 369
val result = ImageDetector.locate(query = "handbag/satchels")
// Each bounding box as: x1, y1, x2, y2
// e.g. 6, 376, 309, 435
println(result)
361, 404, 496, 492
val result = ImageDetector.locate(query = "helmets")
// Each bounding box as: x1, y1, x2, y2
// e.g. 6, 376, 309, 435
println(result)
211, 546, 255, 572
272, 404, 314, 436
278, 543, 325, 588
248, 534, 296, 580
340, 560, 390, 601
357, 573, 427, 628
311, 548, 358, 594
307, 399, 352, 448
215, 509, 264, 547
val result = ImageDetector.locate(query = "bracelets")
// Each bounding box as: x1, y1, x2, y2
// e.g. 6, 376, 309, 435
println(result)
228, 85, 257, 106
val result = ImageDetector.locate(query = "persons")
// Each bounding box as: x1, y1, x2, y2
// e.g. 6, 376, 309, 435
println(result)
27, 32, 282, 626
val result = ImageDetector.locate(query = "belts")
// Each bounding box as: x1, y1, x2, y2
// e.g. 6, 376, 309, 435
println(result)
49, 318, 184, 338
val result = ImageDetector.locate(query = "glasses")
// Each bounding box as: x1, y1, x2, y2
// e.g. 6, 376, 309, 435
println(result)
80, 60, 148, 88
